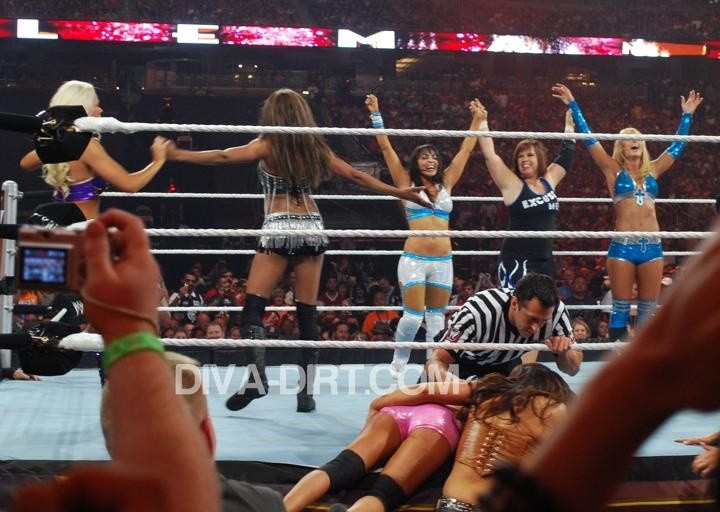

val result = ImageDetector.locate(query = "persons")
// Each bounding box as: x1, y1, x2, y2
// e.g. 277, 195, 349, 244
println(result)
96, 348, 288, 512
153, 85, 437, 414
1, 207, 224, 510
367, 361, 586, 511
671, 429, 719, 480
273, 391, 469, 511
1, 3, 718, 392
476, 224, 720, 512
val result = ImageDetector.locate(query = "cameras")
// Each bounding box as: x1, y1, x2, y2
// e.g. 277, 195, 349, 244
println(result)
13, 225, 85, 291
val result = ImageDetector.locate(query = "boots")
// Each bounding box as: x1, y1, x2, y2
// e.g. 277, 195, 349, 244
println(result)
225, 325, 268, 412
297, 348, 316, 413
